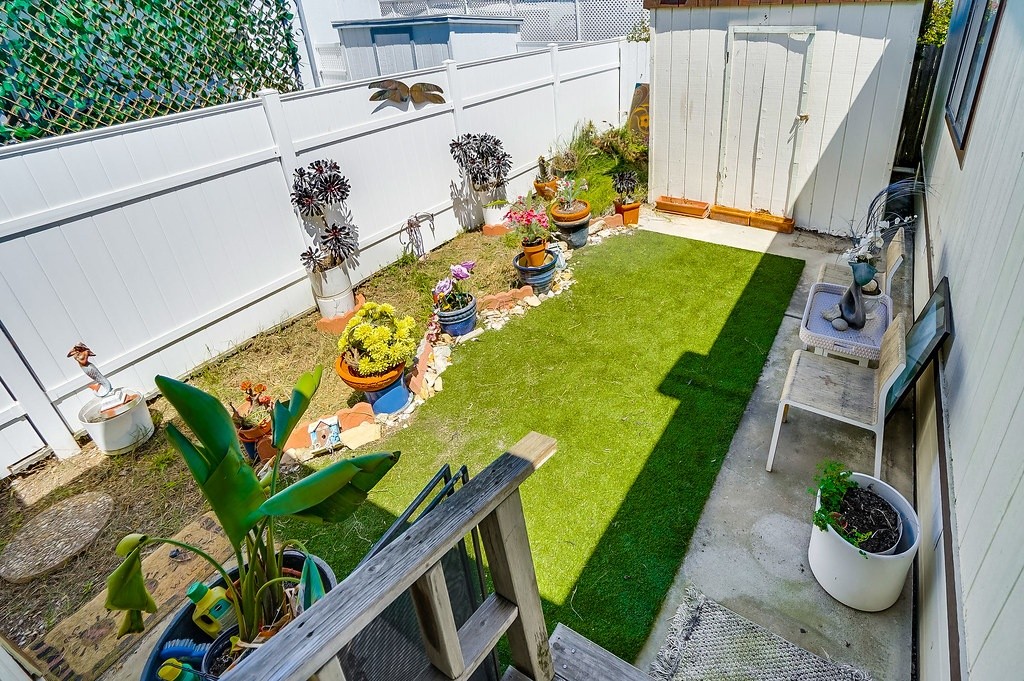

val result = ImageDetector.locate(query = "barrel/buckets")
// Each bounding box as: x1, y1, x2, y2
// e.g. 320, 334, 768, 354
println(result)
808, 472, 920, 613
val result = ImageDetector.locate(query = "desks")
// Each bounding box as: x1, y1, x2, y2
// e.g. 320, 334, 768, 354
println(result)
800, 282, 893, 368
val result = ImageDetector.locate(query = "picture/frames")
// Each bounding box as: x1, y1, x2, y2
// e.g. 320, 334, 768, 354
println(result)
883, 276, 949, 425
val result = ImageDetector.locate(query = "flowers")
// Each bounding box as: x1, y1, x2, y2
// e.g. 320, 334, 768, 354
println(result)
484, 191, 551, 240
344, 302, 419, 377
839, 171, 943, 268
235, 377, 277, 432
546, 177, 594, 209
433, 259, 476, 311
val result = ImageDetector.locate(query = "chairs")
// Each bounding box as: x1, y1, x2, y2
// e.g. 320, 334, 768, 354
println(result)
766, 317, 906, 481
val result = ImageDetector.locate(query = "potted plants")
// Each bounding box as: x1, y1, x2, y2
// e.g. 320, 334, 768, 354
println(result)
448, 135, 512, 225
550, 146, 577, 177
809, 460, 921, 611
106, 372, 403, 681
610, 170, 642, 225
533, 157, 562, 201
289, 160, 357, 320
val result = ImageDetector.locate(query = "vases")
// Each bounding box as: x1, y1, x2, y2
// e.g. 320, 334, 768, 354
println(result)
751, 212, 795, 234
552, 213, 590, 250
77, 386, 154, 455
335, 351, 405, 390
237, 427, 260, 461
513, 249, 559, 293
521, 238, 545, 265
434, 292, 477, 338
708, 203, 752, 227
838, 262, 878, 330
233, 397, 273, 439
654, 195, 709, 219
364, 372, 414, 417
552, 199, 590, 221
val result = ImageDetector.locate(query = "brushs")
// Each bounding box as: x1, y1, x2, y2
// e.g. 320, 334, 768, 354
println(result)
161, 638, 196, 659
189, 643, 212, 663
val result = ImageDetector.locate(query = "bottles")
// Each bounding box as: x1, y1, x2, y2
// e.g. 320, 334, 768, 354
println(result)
182, 581, 238, 640
158, 658, 201, 681
